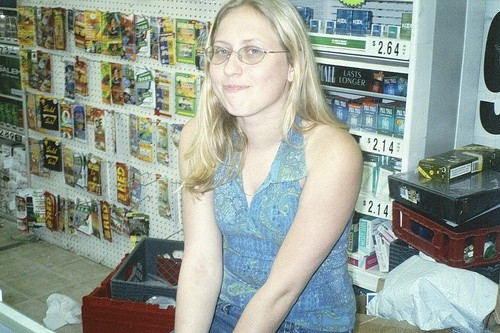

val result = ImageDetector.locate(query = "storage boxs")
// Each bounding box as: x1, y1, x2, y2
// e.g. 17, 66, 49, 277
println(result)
81, 254, 182, 333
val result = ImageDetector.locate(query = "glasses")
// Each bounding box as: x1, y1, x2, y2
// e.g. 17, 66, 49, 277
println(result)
204, 45, 292, 65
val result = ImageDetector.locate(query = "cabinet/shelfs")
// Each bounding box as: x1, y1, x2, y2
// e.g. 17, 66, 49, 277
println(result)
287, 0, 484, 295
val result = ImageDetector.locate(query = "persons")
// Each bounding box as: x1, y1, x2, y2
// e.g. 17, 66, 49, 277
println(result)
172, 0, 362, 333
